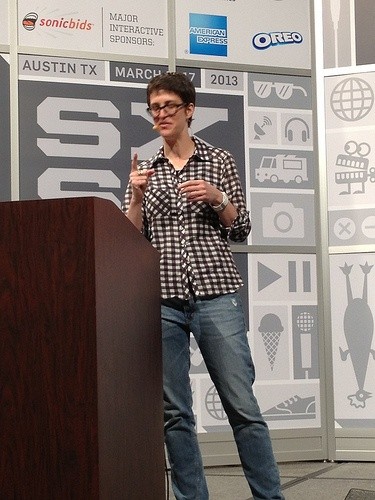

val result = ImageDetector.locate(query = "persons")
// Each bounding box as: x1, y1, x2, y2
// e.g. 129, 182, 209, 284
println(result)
119, 72, 284, 500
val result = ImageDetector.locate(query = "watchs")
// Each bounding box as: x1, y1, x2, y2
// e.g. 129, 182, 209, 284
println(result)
208, 191, 228, 212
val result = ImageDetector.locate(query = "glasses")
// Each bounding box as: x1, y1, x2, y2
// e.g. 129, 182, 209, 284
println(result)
146, 103, 186, 118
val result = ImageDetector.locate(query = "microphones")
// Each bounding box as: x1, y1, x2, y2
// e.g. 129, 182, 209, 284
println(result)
152, 124, 158, 131
